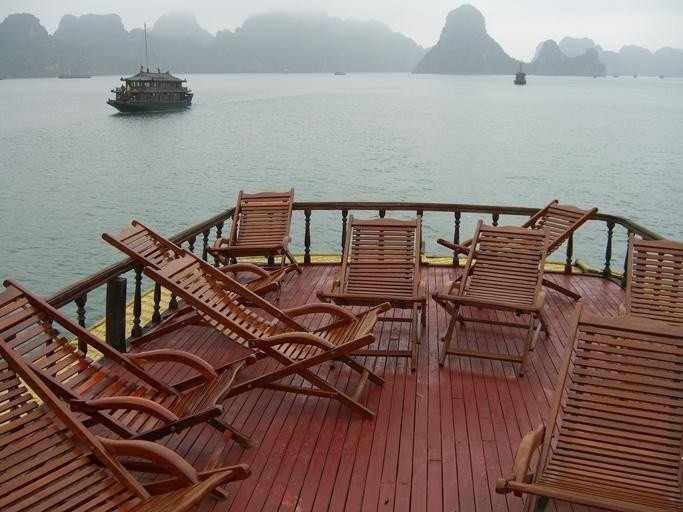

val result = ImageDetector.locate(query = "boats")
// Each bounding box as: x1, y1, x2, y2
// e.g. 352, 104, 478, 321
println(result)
486, 56, 528, 89
106, 22, 195, 112
58, 72, 94, 80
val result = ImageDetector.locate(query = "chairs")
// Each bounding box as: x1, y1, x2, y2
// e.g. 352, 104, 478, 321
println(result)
495, 304, 683, 512
603, 230, 682, 386
315, 212, 428, 371
204, 187, 304, 301
139, 246, 391, 421
0, 333, 255, 511
101, 217, 300, 347
1, 273, 256, 501
437, 198, 600, 304
432, 218, 551, 380
